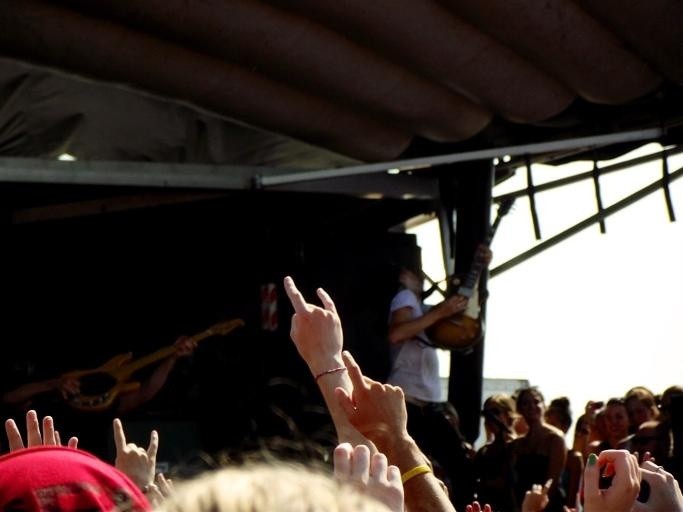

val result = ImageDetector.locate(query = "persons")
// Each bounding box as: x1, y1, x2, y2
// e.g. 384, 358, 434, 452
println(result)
331, 350, 456, 512
384, 240, 494, 404
281, 276, 377, 447
9, 333, 197, 409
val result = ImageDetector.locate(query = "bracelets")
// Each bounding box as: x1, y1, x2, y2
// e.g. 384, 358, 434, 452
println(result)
309, 367, 345, 377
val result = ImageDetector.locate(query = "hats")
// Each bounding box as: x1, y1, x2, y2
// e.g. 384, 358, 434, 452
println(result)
479, 393, 522, 420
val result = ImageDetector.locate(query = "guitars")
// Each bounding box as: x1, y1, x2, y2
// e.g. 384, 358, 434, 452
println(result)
425, 196, 519, 353
69, 320, 241, 412
